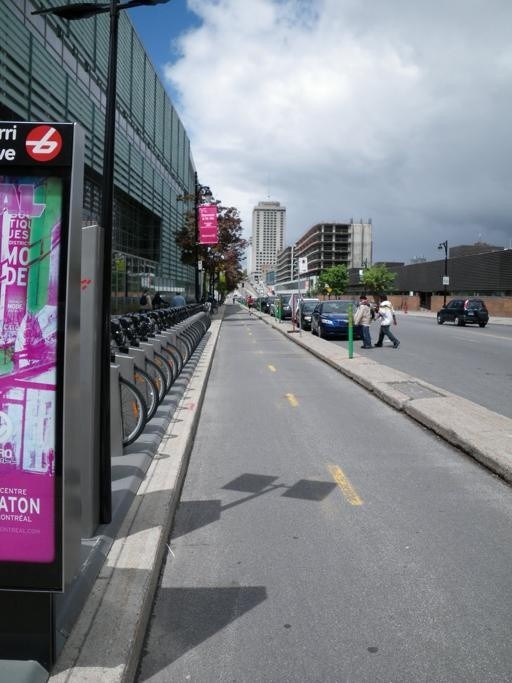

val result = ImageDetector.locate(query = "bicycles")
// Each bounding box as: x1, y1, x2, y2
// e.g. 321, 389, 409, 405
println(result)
110, 302, 211, 448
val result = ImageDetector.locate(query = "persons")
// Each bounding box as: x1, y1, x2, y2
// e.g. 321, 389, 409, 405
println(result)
151, 290, 168, 310
354, 296, 375, 348
233, 297, 235, 304
170, 291, 187, 307
139, 289, 153, 310
375, 295, 400, 349
247, 295, 254, 311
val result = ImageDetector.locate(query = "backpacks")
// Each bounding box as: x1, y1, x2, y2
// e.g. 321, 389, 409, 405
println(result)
140, 294, 148, 306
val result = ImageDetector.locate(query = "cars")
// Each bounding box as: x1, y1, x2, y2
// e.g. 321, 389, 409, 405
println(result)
234, 295, 360, 338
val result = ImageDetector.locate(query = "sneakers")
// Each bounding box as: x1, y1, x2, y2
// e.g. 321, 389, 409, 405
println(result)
361, 341, 400, 348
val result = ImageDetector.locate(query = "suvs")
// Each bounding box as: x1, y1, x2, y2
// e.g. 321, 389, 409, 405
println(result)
437, 298, 489, 328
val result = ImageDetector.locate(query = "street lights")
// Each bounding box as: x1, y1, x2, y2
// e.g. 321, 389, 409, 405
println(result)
437, 240, 448, 306
